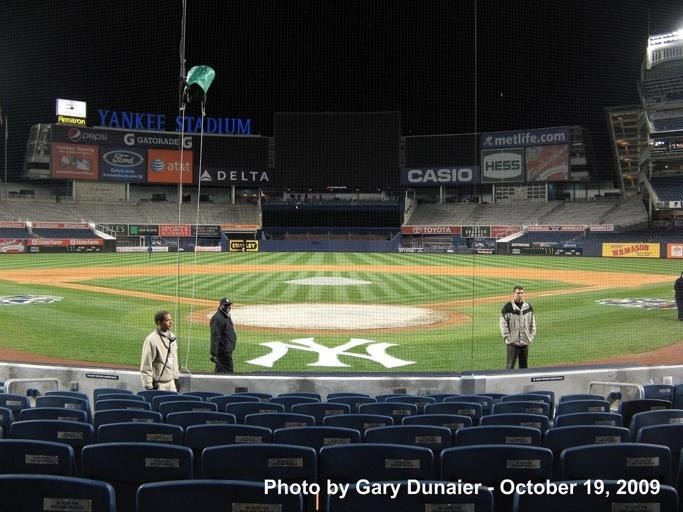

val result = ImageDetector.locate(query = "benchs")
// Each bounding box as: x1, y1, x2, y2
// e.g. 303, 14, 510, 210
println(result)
400, 200, 650, 226
0, 196, 259, 239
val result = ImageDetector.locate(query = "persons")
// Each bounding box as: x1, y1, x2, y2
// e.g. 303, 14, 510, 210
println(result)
147, 246, 152, 258
674, 271, 683, 321
140, 311, 180, 393
500, 284, 536, 369
209, 297, 237, 373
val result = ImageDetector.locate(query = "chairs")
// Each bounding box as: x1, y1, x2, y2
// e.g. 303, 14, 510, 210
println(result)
637, 71, 683, 205
422, 228, 683, 261
0, 377, 683, 512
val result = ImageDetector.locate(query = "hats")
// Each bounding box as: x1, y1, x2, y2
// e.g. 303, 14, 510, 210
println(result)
220, 298, 233, 306
